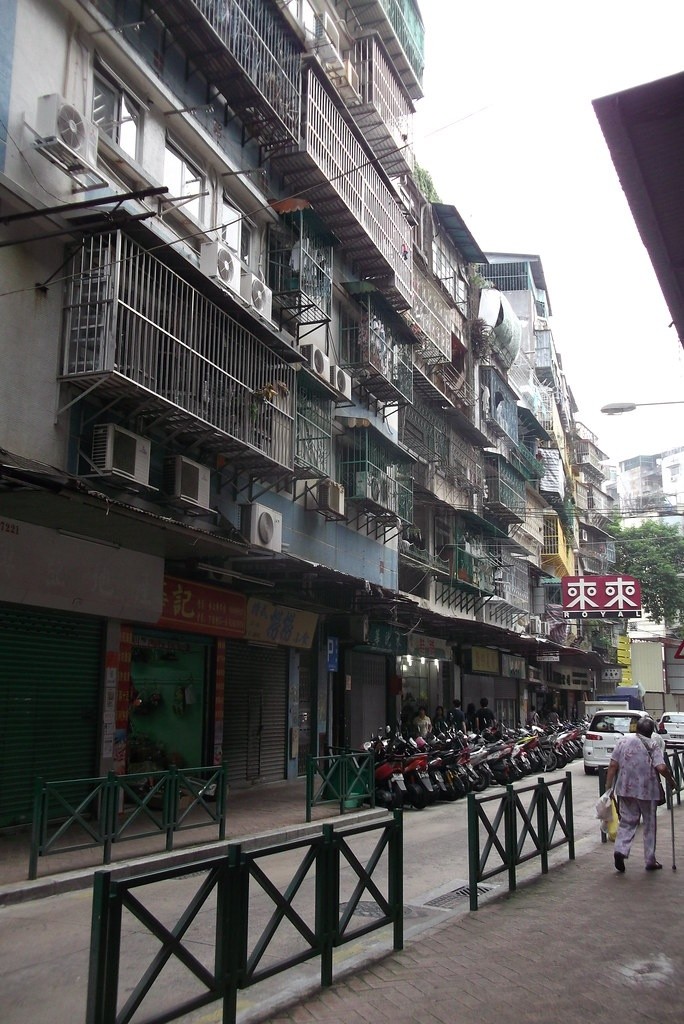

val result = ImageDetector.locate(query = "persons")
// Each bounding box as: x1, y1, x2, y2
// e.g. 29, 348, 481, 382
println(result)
604, 717, 680, 872
475, 697, 496, 732
412, 706, 432, 738
527, 704, 540, 728
432, 706, 446, 734
446, 698, 467, 734
546, 703, 560, 725
464, 702, 479, 732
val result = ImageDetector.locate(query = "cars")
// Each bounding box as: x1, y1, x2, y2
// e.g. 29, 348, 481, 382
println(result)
580, 709, 660, 777
656, 711, 684, 750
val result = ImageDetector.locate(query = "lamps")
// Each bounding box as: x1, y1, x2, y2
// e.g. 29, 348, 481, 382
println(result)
356, 471, 380, 503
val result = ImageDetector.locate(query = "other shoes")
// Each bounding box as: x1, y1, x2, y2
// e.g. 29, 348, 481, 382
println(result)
614, 851, 626, 872
646, 861, 662, 870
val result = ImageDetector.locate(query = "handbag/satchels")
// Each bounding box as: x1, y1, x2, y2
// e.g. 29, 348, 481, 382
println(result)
529, 712, 538, 726
596, 788, 620, 842
656, 777, 666, 806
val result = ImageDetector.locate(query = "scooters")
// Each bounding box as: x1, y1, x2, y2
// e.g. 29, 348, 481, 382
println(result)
357, 710, 594, 814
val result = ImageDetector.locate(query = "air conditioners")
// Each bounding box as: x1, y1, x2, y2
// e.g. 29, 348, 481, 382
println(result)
312, 10, 340, 64
89, 422, 152, 488
529, 618, 551, 636
335, 59, 359, 102
239, 501, 282, 553
161, 454, 210, 510
344, 615, 370, 643
36, 92, 99, 175
317, 478, 344, 516
579, 528, 588, 542
199, 241, 273, 320
300, 343, 352, 401
